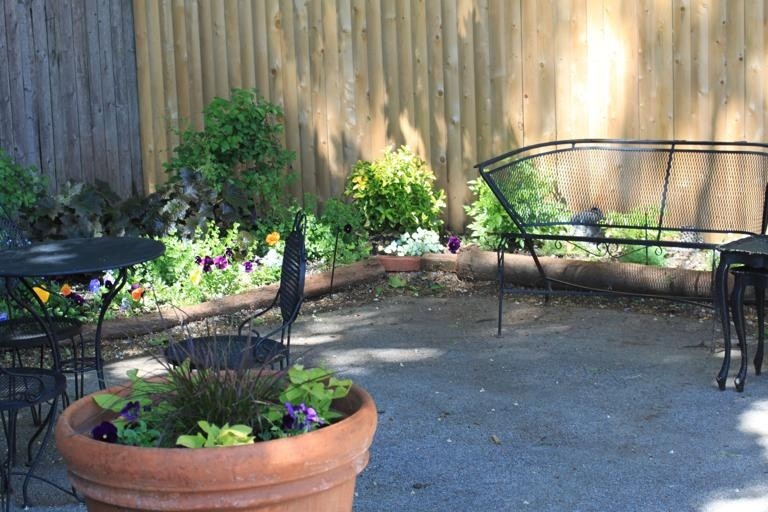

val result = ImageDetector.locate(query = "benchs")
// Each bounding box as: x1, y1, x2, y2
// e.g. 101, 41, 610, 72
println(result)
472, 136, 768, 336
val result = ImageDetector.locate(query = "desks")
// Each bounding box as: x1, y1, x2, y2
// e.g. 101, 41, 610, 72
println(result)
0, 235, 165, 412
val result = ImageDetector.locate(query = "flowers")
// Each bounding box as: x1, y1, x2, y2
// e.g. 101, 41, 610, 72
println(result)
82, 299, 353, 452
414, 226, 448, 254
382, 232, 421, 257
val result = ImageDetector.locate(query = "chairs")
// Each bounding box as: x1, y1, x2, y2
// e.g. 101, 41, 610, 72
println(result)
0, 203, 83, 427
711, 234, 768, 393
166, 210, 307, 371
1, 348, 84, 512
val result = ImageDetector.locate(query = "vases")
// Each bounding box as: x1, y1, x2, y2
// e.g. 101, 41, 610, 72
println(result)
421, 253, 458, 274
52, 374, 377, 512
378, 256, 421, 279
